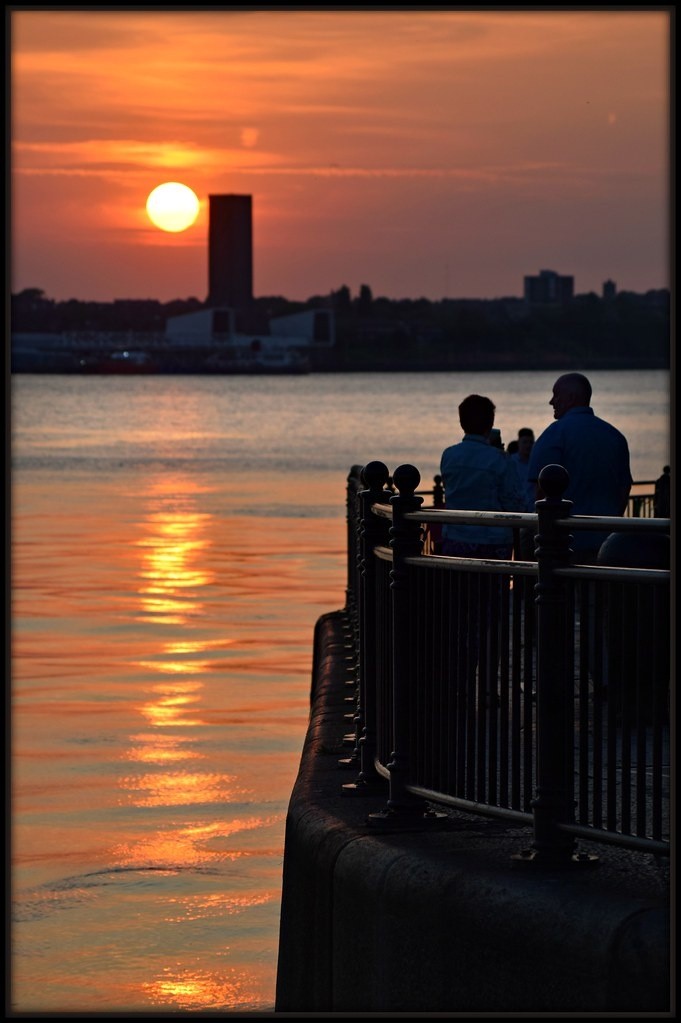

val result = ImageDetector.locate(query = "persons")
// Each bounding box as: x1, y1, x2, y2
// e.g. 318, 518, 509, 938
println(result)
525, 372, 634, 699
440, 394, 529, 706
